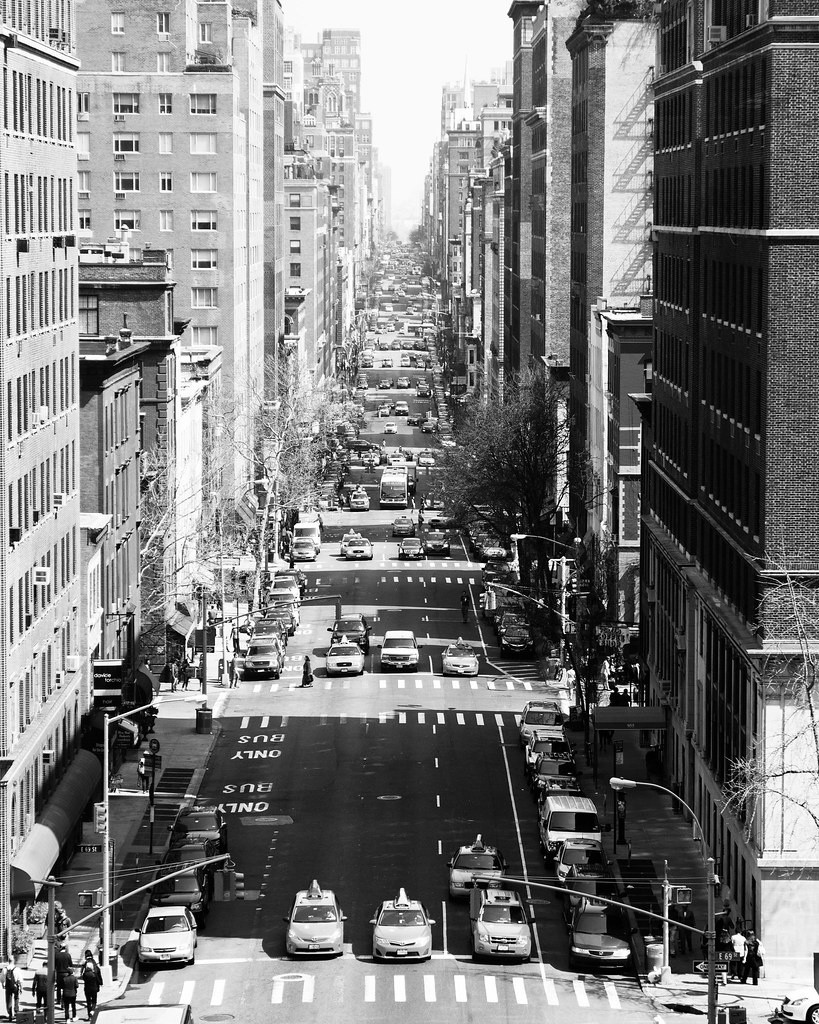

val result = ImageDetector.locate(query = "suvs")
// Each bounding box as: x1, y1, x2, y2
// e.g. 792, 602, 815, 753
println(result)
560, 863, 621, 921
160, 836, 216, 883
518, 699, 587, 816
167, 804, 228, 868
565, 894, 640, 974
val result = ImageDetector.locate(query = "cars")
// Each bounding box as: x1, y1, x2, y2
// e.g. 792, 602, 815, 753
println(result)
133, 905, 198, 971
368, 887, 437, 963
552, 837, 614, 886
446, 833, 507, 899
281, 878, 348, 959
146, 863, 210, 928
236, 248, 536, 680
469, 887, 536, 964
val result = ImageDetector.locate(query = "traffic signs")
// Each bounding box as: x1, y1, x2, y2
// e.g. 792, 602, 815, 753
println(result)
693, 959, 729, 974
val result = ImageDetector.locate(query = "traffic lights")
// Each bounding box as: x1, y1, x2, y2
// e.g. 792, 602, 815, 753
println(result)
229, 870, 245, 902
94, 803, 107, 832
78, 892, 93, 909
674, 887, 693, 905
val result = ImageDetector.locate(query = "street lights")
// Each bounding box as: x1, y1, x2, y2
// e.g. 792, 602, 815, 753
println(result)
609, 776, 715, 1024
510, 533, 582, 706
217, 477, 269, 689
94, 693, 209, 989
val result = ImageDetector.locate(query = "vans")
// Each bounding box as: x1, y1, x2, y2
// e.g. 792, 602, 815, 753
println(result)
536, 795, 602, 867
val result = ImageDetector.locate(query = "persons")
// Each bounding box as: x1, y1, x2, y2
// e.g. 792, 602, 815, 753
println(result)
407, 912, 423, 924
0, 901, 104, 1024
398, 447, 437, 531
146, 568, 249, 693
321, 909, 336, 920
280, 429, 386, 560
137, 751, 150, 794
460, 590, 470, 623
556, 653, 643, 754
298, 655, 313, 688
669, 901, 764, 985
